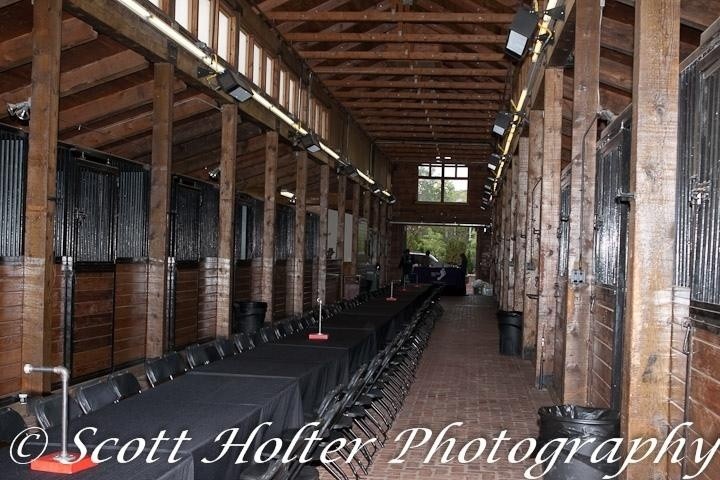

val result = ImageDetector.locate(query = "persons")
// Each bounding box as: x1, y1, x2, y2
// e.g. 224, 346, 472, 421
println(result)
458, 253, 468, 274
327, 247, 336, 259
398, 249, 430, 282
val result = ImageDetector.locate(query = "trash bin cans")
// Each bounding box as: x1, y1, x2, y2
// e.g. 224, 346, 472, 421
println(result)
232, 301, 268, 333
532, 405, 621, 480
495, 310, 523, 356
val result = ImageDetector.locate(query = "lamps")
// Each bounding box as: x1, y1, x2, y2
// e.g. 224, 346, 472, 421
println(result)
297, 131, 396, 205
477, 109, 519, 211
505, 3, 566, 60
6, 96, 30, 122
215, 66, 254, 103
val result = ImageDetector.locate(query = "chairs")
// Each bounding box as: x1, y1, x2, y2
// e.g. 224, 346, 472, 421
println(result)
0, 278, 444, 480
